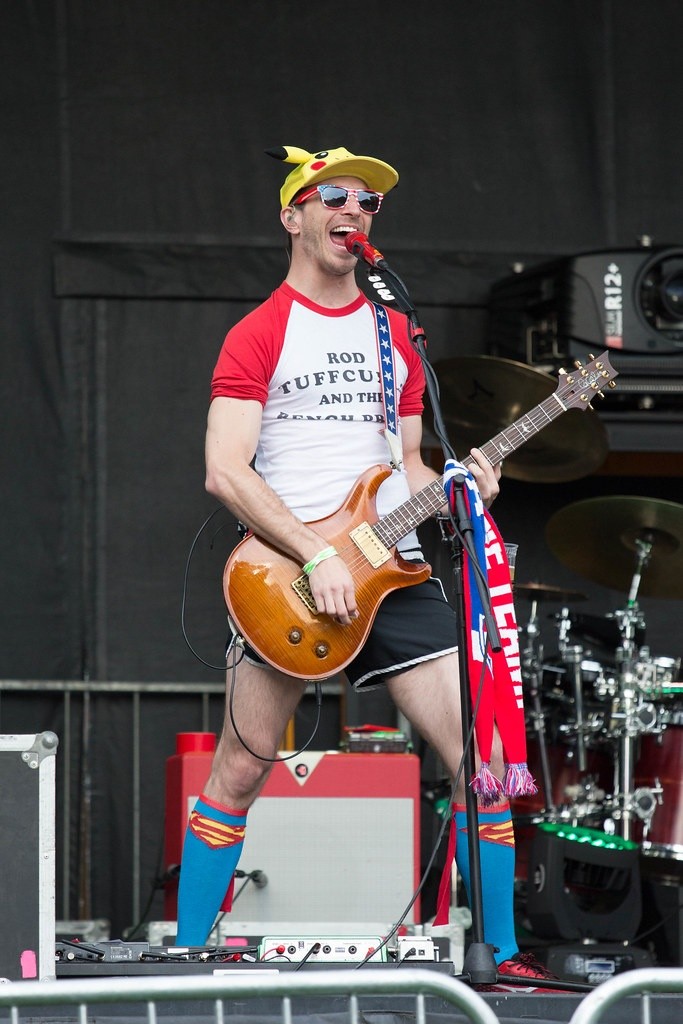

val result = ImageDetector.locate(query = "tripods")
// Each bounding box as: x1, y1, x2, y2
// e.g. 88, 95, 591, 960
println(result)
370, 263, 597, 994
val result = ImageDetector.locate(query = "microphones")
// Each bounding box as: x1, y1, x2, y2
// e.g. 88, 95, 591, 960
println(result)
344, 231, 388, 271
250, 870, 268, 888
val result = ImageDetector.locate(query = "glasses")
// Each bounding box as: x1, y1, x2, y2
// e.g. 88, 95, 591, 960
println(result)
290, 186, 384, 214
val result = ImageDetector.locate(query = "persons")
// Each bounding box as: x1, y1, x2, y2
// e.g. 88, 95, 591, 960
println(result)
173, 146, 579, 999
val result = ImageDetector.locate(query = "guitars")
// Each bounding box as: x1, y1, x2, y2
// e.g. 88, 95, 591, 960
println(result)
215, 344, 624, 686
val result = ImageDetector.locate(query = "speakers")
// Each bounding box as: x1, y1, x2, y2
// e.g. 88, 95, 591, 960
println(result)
167, 752, 421, 947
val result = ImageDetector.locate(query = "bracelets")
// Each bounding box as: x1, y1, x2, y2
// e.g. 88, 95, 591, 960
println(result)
302, 545, 339, 577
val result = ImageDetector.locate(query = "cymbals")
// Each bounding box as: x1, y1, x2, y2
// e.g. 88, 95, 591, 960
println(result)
540, 491, 683, 606
513, 579, 592, 605
426, 351, 615, 487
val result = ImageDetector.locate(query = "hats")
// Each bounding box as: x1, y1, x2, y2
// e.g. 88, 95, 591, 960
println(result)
264, 145, 399, 210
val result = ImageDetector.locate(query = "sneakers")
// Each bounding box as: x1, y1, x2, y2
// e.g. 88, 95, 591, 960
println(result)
472, 952, 579, 993
